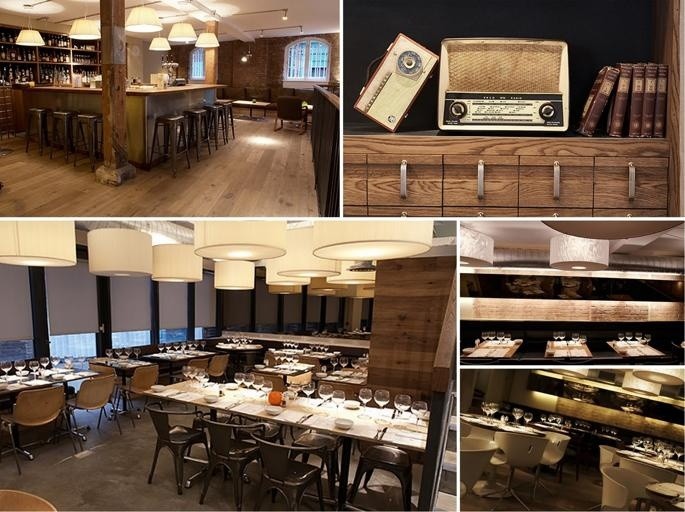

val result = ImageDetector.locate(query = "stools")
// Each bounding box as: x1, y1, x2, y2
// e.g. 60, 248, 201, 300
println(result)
24, 105, 104, 173
149, 98, 247, 178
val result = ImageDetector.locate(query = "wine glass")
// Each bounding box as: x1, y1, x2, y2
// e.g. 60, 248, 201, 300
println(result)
158, 340, 207, 354
553, 331, 587, 348
480, 330, 512, 347
0, 347, 142, 390
630, 436, 685, 467
225, 335, 254, 348
271, 339, 369, 381
478, 400, 617, 436
618, 332, 652, 348
180, 364, 428, 429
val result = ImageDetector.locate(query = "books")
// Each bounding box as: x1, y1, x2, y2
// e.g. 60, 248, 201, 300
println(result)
627, 63, 645, 138
651, 63, 669, 137
639, 62, 659, 138
575, 64, 619, 136
606, 62, 633, 137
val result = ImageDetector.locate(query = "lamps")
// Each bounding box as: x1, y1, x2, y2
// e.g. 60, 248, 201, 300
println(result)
125, 0, 220, 52
621, 369, 685, 398
461, 226, 613, 269
15, 2, 102, 46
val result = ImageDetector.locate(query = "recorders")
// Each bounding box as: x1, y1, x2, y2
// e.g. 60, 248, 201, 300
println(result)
436, 37, 570, 137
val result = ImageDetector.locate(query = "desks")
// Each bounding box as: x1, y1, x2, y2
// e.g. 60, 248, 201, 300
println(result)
233, 98, 270, 117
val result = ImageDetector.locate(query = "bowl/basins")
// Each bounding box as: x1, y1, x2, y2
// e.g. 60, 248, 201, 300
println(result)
151, 384, 166, 393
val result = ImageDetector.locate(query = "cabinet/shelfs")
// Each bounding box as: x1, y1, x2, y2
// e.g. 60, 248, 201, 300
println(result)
0, 22, 100, 86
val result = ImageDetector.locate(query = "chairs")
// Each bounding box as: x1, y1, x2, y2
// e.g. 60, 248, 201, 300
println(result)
461, 420, 684, 511
273, 97, 308, 136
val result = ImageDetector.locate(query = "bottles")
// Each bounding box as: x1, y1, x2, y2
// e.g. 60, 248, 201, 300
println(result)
0, 28, 101, 89
